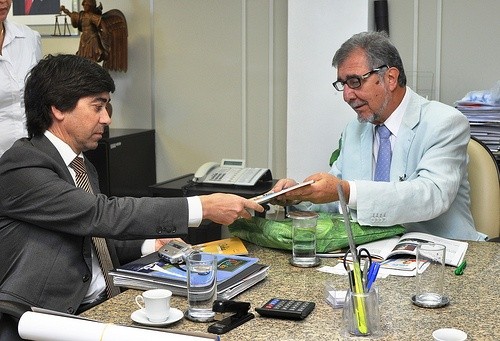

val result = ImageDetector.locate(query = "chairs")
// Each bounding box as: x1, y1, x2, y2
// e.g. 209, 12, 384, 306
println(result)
467, 135, 500, 243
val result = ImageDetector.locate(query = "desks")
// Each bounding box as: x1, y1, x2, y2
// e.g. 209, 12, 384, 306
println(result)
146, 173, 280, 245
78, 239, 500, 341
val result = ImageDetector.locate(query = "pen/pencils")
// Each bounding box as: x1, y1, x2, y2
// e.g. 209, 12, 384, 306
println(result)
362, 260, 380, 293
353, 262, 368, 334
347, 264, 356, 315
455, 260, 467, 275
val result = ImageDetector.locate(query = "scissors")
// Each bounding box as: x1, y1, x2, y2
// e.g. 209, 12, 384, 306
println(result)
343, 248, 372, 276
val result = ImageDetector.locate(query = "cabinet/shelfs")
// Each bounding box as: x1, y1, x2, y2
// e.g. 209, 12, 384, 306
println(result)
82, 128, 156, 196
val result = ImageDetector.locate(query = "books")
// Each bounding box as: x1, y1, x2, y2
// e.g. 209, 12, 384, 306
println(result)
356, 232, 469, 268
108, 237, 270, 300
241, 180, 314, 208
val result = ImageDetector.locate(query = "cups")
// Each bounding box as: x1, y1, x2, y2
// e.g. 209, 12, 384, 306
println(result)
186, 252, 218, 320
415, 243, 446, 306
431, 328, 467, 341
135, 288, 172, 322
292, 215, 318, 265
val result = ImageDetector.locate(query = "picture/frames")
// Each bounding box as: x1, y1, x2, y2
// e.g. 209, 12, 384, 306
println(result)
6, 0, 79, 36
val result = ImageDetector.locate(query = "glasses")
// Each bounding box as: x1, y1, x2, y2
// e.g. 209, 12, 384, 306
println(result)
332, 64, 391, 91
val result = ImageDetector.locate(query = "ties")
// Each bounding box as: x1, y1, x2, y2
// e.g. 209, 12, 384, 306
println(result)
68, 156, 120, 300
374, 125, 392, 182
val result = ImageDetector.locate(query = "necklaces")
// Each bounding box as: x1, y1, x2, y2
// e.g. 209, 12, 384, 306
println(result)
1, 30, 3, 33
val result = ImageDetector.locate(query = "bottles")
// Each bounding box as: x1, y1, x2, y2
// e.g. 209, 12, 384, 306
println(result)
342, 289, 380, 336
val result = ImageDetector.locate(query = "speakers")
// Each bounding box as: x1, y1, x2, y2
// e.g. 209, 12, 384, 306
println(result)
80, 127, 156, 202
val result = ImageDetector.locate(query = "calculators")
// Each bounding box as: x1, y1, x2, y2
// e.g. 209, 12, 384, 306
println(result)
254, 299, 315, 320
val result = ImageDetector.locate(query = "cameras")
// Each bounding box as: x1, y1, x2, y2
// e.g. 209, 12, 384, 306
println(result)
156, 239, 202, 266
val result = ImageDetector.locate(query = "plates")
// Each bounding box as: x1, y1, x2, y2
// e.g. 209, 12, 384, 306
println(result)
130, 307, 184, 326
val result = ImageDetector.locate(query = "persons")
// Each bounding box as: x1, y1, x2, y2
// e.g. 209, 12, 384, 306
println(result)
0, 0, 42, 153
0, 54, 264, 341
270, 32, 486, 243
60, 0, 111, 73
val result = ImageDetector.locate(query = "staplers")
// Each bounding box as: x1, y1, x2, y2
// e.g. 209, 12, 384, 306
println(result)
207, 299, 255, 335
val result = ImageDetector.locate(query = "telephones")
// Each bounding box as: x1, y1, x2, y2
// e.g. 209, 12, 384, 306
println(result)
192, 159, 273, 187
